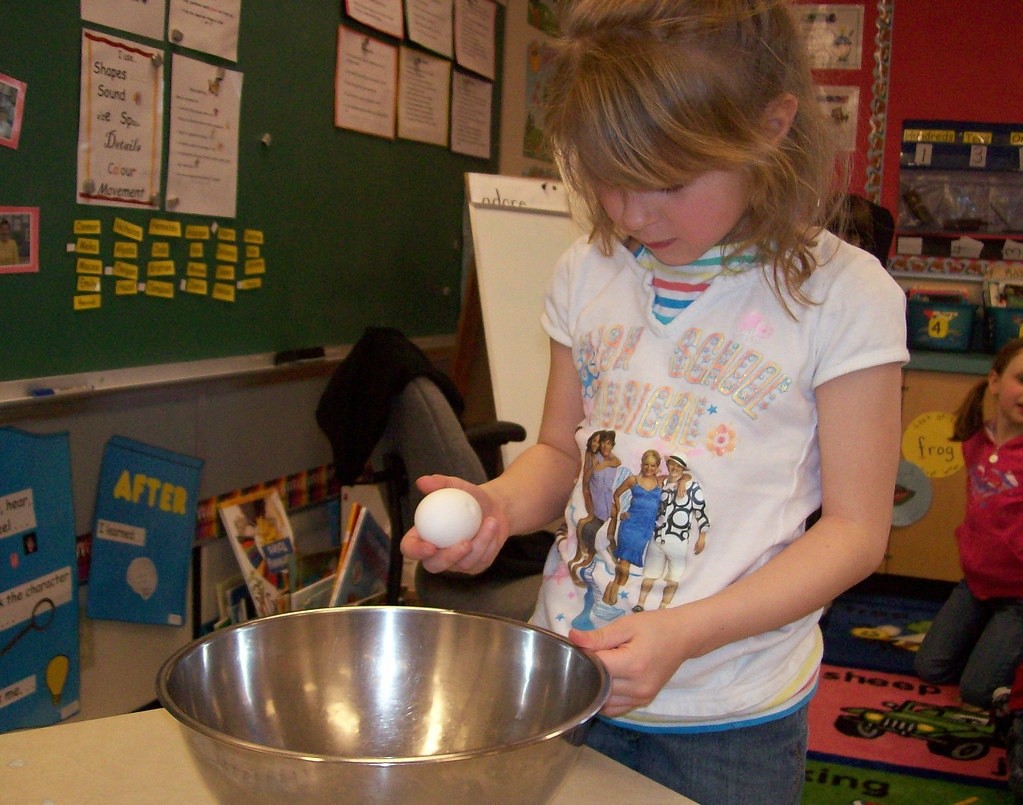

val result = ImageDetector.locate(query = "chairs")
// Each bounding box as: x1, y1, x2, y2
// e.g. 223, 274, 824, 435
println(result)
315, 326, 554, 622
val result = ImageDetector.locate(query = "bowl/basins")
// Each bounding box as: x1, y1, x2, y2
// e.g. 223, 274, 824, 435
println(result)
156, 605, 612, 805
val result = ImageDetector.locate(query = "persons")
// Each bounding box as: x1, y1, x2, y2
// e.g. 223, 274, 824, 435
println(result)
400, 0, 911, 805
566, 431, 710, 612
1, 219, 20, 264
913, 335, 1023, 707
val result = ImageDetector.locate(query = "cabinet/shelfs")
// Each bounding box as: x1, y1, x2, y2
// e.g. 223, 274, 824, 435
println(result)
864, 223, 1023, 582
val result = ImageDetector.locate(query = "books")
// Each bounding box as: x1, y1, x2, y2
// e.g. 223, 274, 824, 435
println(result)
327, 504, 391, 608
213, 574, 255, 631
217, 487, 296, 618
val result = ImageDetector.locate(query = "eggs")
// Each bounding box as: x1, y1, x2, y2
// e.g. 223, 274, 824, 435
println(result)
413, 487, 482, 548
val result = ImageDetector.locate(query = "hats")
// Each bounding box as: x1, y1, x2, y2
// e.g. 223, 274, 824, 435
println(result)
664, 452, 689, 472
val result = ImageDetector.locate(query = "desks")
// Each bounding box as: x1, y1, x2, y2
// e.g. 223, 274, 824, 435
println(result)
0, 709, 697, 805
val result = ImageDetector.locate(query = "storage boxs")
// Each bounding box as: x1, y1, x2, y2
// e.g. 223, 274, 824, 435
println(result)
904, 288, 1023, 356
